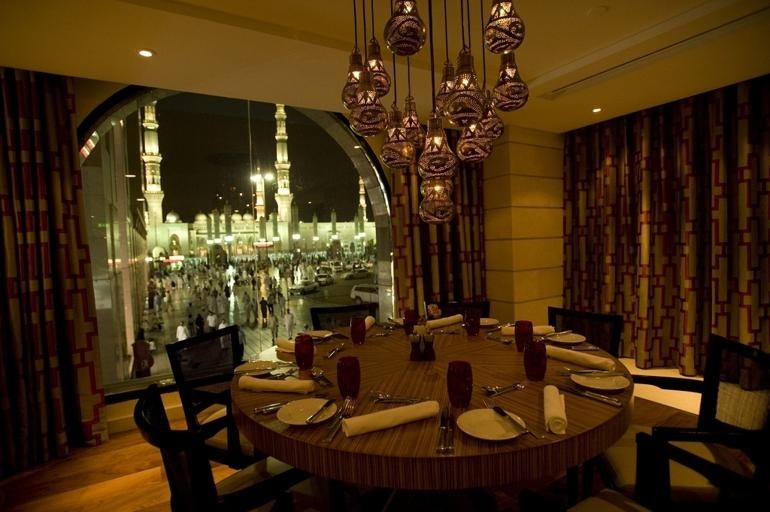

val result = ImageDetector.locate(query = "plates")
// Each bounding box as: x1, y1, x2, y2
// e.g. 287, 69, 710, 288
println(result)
276, 397, 337, 425
548, 333, 586, 343
234, 360, 278, 377
480, 317, 500, 326
571, 370, 630, 392
457, 409, 526, 439
308, 330, 332, 337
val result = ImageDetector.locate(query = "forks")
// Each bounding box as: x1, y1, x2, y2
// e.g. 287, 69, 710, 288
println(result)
325, 396, 356, 442
376, 317, 402, 337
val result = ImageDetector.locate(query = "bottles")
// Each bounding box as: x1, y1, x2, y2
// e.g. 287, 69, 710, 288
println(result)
409, 334, 436, 362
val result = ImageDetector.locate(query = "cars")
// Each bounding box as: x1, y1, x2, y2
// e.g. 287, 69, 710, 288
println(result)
341, 268, 371, 280
317, 273, 333, 286
317, 258, 369, 272
289, 278, 318, 295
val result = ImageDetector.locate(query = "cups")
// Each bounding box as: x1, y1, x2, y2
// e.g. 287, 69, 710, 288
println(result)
350, 316, 367, 343
463, 310, 480, 337
295, 334, 313, 370
448, 360, 473, 408
404, 311, 417, 336
336, 356, 360, 397
515, 319, 534, 351
525, 339, 548, 382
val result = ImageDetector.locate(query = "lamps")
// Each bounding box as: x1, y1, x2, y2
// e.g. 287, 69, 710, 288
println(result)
434, 1, 462, 124
484, 1, 526, 57
379, 53, 416, 172
443, 1, 490, 127
350, 2, 390, 136
341, 1, 378, 111
492, 54, 528, 112
418, 180, 456, 197
454, 2, 493, 163
400, 53, 425, 155
363, 2, 392, 98
382, 1, 428, 58
473, 1, 505, 143
417, 181, 457, 223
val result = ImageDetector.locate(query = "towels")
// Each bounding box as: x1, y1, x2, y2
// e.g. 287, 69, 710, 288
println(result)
501, 326, 552, 335
427, 314, 463, 329
363, 315, 375, 330
275, 337, 295, 350
546, 345, 616, 372
238, 375, 315, 395
542, 385, 567, 436
342, 400, 439, 437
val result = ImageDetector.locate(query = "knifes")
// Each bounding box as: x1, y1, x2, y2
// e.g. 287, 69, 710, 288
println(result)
437, 407, 455, 454
493, 406, 539, 441
253, 392, 330, 414
562, 385, 622, 409
306, 398, 335, 422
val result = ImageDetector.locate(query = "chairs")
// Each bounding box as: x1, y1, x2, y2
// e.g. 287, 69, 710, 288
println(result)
165, 325, 261, 468
608, 333, 770, 502
567, 430, 755, 512
310, 303, 375, 330
548, 307, 624, 360
134, 385, 315, 512
424, 299, 490, 321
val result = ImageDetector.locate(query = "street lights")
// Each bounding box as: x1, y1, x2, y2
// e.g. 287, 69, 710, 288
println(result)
312, 237, 320, 256
273, 236, 281, 264
258, 236, 266, 264
327, 230, 366, 258
249, 159, 274, 277
214, 237, 222, 265
207, 239, 214, 265
225, 236, 234, 266
292, 234, 301, 255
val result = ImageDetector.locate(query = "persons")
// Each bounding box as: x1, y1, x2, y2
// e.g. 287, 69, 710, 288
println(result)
132, 328, 151, 378
155, 252, 366, 346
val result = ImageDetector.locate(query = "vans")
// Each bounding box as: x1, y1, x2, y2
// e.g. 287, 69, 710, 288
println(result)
350, 283, 379, 306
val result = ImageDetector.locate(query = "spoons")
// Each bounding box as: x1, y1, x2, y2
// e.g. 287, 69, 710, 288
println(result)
486, 333, 512, 344
310, 366, 332, 386
552, 365, 605, 376
327, 342, 344, 358
370, 389, 431, 401
536, 329, 572, 342
480, 382, 524, 396
